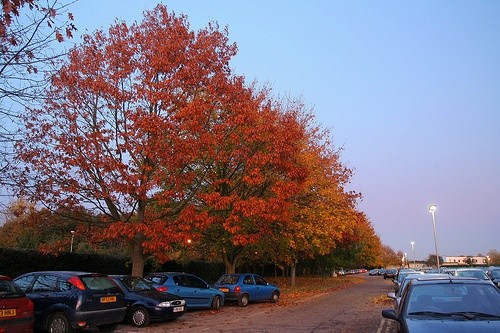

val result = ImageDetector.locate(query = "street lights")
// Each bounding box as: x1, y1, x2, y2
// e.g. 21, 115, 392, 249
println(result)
401, 252, 407, 269
69, 230, 76, 252
411, 241, 418, 268
428, 203, 440, 274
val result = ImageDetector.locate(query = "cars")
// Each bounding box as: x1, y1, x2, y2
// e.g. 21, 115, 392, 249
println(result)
336, 266, 367, 276
381, 266, 500, 333
0, 276, 33, 333
214, 273, 281, 305
389, 268, 399, 277
384, 271, 396, 279
109, 274, 186, 326
143, 272, 225, 310
368, 268, 386, 276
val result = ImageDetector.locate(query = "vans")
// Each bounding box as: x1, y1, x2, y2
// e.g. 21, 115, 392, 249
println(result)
14, 271, 126, 332
